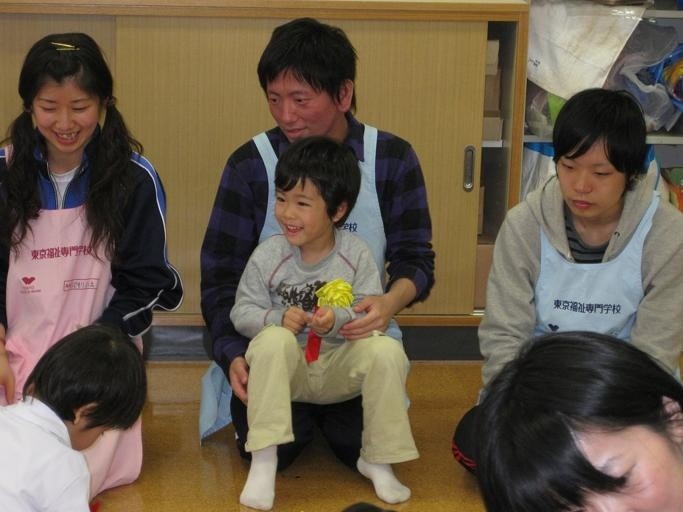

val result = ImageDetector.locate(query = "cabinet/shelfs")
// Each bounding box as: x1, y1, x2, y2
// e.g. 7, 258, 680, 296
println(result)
0, 0, 529, 330
479, 1, 682, 243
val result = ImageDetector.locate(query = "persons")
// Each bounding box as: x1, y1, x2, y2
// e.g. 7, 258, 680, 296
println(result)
0, 32, 184, 504
451, 88, 683, 480
474, 330, 682, 512
229, 135, 421, 512
198, 17, 435, 471
0, 326, 145, 512
